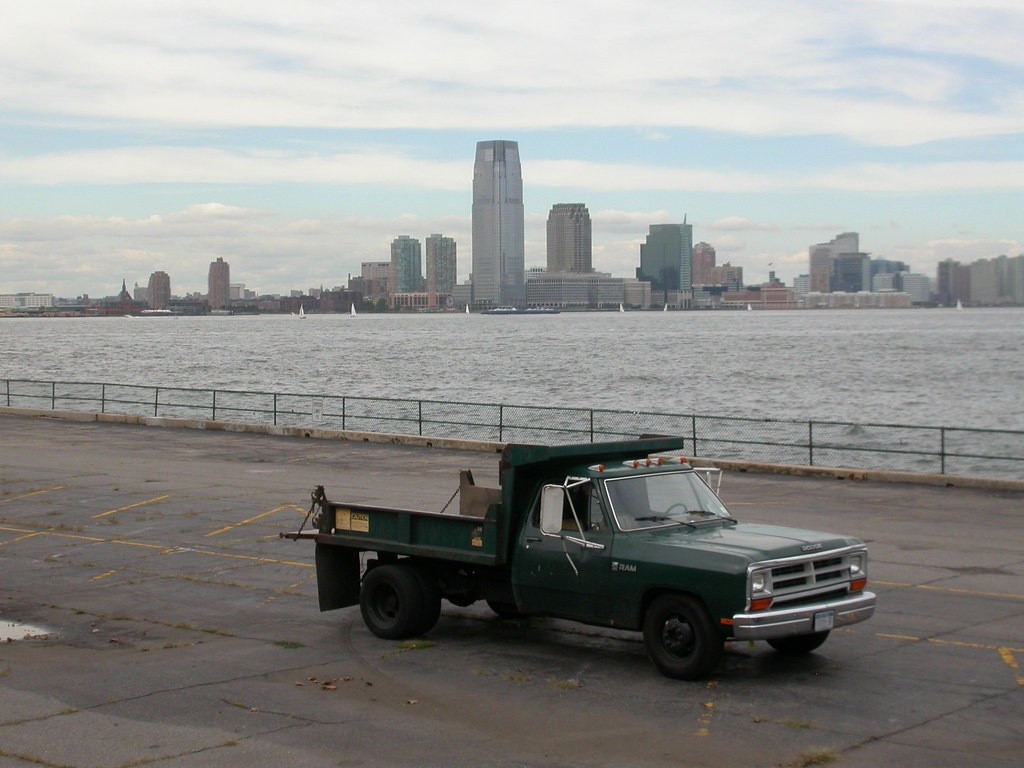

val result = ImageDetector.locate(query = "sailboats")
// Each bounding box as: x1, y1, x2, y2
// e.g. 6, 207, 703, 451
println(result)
663, 304, 668, 312
298, 302, 307, 319
466, 304, 470, 314
619, 302, 625, 313
350, 303, 357, 317
955, 298, 964, 312
747, 304, 753, 311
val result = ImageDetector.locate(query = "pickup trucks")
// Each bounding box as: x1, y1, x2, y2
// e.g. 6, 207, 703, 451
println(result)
279, 434, 878, 680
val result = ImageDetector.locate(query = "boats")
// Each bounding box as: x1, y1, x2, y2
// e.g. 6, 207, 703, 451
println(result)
124, 315, 140, 319
289, 311, 296, 318
480, 306, 562, 315
